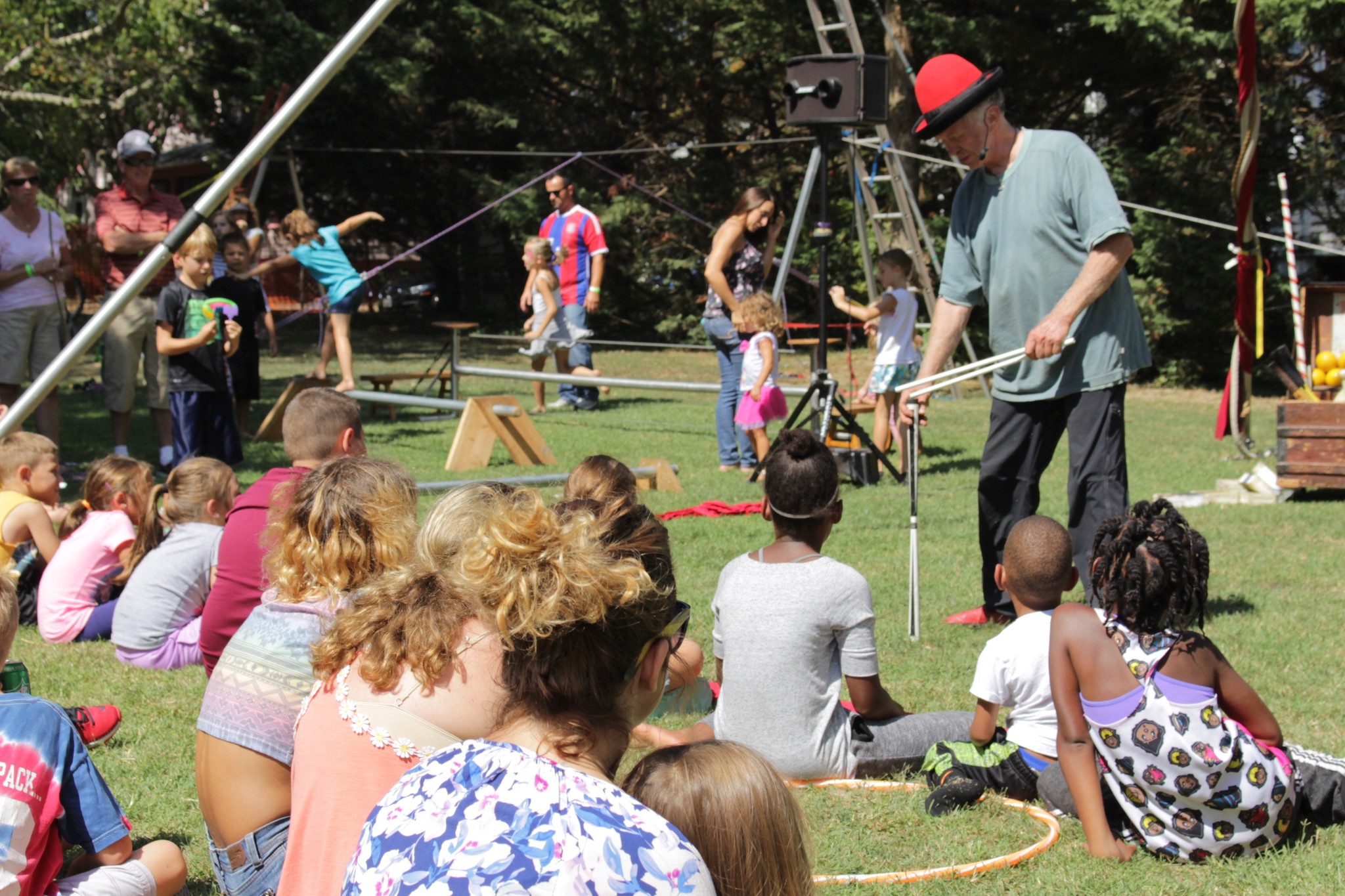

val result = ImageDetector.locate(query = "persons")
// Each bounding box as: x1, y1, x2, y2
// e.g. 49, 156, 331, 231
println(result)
1036, 498, 1345, 864
196, 456, 420, 896
0, 406, 239, 672
565, 456, 712, 722
339, 475, 715, 896
0, 130, 275, 474
229, 208, 387, 394
922, 513, 1078, 817
629, 428, 975, 782
701, 184, 788, 484
276, 479, 528, 896
198, 389, 366, 676
62, 704, 122, 749
898, 55, 1153, 626
0, 576, 188, 896
519, 171, 610, 413
621, 741, 814, 896
827, 249, 924, 477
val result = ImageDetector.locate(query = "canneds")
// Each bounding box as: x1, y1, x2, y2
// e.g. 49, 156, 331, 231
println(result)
0, 660, 31, 695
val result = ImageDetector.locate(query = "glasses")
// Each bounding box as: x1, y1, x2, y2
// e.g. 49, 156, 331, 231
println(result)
624, 600, 690, 682
7, 176, 38, 186
120, 156, 154, 167
546, 185, 569, 197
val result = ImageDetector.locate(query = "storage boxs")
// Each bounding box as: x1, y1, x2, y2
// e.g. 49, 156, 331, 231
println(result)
1267, 278, 1345, 493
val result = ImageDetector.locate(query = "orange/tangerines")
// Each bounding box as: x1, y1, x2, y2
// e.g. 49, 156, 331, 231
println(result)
1312, 351, 1345, 386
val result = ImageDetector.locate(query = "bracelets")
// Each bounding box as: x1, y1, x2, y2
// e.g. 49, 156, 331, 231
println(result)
25, 263, 33, 278
589, 286, 600, 293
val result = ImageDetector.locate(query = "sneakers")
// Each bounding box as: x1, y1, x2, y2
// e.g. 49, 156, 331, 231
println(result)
63, 705, 122, 750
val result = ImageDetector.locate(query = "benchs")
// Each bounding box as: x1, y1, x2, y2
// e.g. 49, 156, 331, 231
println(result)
825, 401, 894, 450
359, 369, 453, 422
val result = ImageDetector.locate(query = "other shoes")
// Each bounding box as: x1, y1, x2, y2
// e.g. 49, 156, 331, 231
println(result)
547, 397, 569, 409
573, 397, 599, 410
157, 460, 173, 478
944, 605, 1011, 625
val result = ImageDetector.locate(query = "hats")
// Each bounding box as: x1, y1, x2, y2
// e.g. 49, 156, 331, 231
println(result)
117, 130, 157, 158
911, 53, 1003, 142
